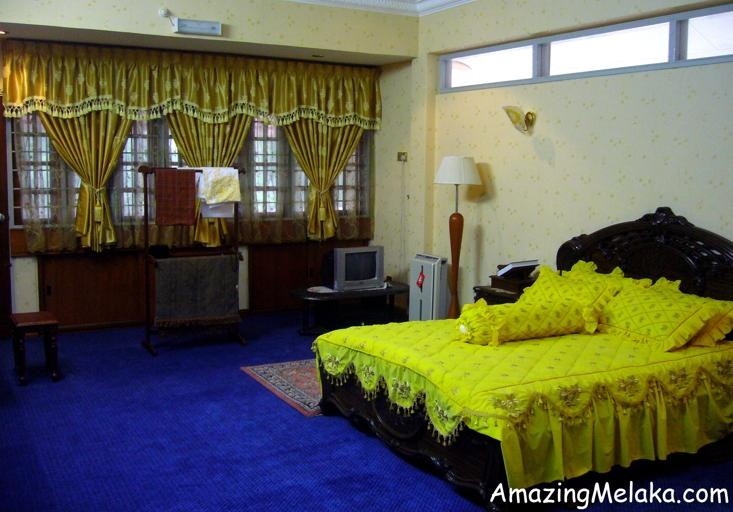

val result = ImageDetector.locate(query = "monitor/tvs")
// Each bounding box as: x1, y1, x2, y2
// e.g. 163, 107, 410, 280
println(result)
320, 245, 384, 291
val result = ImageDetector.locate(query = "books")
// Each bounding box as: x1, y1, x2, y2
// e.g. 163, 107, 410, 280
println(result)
496, 259, 540, 279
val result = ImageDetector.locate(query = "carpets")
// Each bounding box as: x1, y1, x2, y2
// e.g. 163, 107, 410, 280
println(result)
239, 358, 321, 419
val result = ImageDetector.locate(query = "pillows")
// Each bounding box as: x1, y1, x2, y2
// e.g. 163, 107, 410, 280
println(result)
457, 261, 733, 352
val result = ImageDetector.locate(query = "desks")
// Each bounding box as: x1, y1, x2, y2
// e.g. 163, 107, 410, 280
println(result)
289, 281, 408, 336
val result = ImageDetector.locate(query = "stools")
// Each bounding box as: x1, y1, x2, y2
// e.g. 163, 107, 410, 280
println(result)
9, 311, 61, 385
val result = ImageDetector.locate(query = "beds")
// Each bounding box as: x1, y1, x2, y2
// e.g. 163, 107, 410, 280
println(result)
311, 207, 733, 512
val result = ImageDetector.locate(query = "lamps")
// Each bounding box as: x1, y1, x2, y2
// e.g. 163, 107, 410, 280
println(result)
433, 156, 483, 320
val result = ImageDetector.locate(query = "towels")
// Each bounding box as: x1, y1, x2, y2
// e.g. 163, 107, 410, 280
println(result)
154, 163, 240, 228
